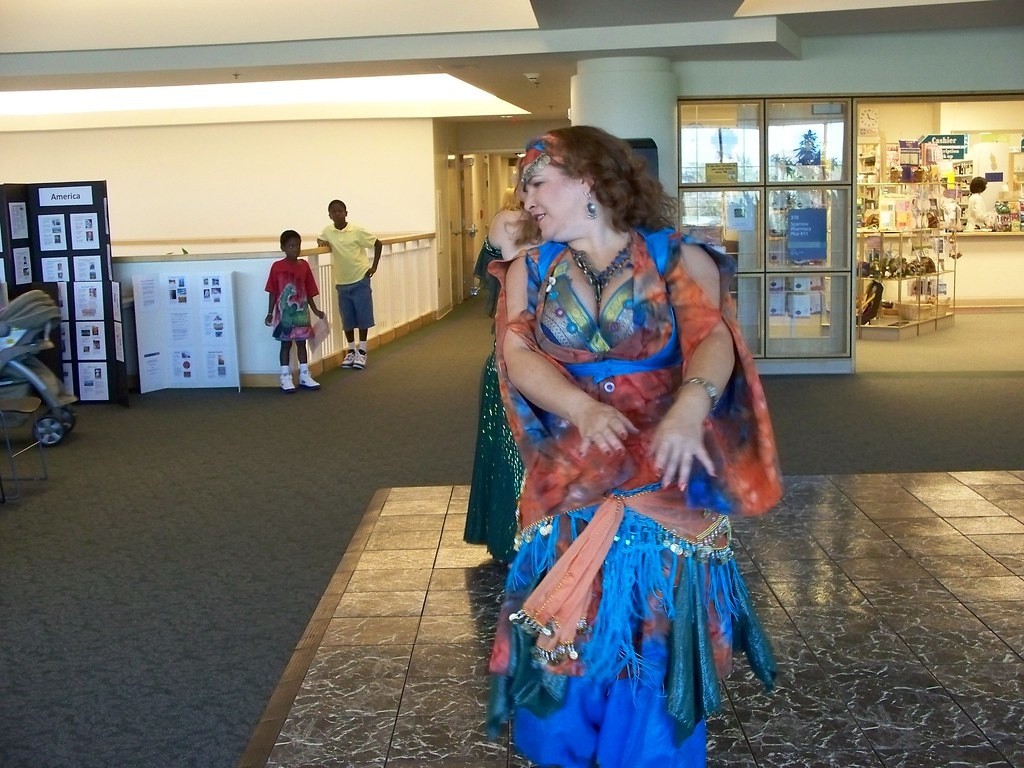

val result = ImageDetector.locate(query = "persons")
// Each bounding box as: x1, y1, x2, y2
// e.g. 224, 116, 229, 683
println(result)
484, 125, 788, 768
264, 229, 324, 393
964, 177, 991, 230
463, 153, 546, 571
317, 200, 382, 369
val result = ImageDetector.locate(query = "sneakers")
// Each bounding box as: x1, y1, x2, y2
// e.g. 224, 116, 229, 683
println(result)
353, 348, 368, 369
341, 349, 356, 369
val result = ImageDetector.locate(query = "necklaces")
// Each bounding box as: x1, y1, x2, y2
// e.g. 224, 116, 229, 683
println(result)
566, 236, 634, 307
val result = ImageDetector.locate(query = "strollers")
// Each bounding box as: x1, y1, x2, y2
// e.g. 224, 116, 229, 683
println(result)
1, 281, 79, 448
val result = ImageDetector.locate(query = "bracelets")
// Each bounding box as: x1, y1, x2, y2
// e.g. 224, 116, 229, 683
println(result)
268, 313, 272, 315
678, 376, 719, 412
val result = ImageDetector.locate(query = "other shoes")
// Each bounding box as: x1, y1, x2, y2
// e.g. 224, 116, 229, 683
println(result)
279, 374, 296, 394
298, 370, 320, 390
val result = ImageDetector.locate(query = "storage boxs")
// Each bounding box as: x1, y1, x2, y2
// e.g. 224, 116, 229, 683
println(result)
770, 239, 821, 318
879, 194, 915, 229
896, 300, 951, 321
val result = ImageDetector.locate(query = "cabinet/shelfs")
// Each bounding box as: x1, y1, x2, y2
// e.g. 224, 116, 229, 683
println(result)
854, 182, 967, 342
678, 191, 829, 335
